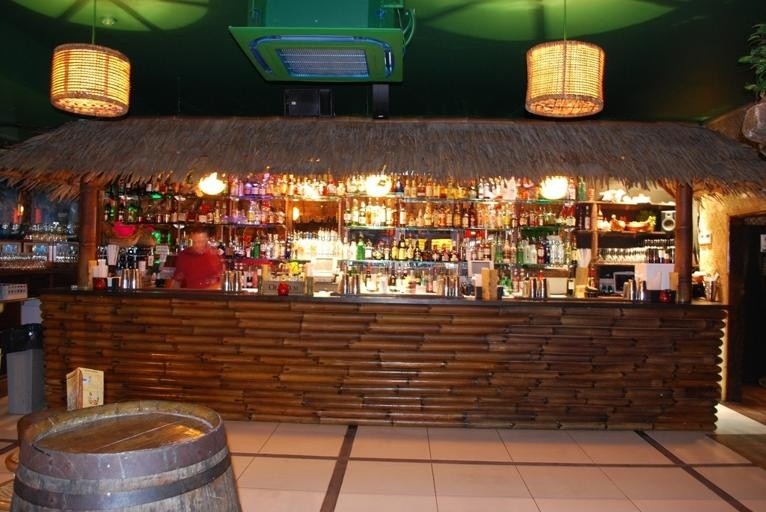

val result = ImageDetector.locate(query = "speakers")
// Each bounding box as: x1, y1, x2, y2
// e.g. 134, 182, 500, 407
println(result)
661, 210, 676, 233
372, 84, 389, 120
283, 87, 334, 118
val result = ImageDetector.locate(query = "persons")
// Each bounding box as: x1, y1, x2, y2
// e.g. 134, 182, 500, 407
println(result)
163, 225, 226, 290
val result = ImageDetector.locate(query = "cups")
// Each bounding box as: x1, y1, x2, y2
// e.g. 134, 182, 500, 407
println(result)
702, 281, 721, 301
565, 271, 576, 297
98, 258, 106, 266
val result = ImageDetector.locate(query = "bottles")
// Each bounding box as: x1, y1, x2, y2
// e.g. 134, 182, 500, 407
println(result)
102, 184, 230, 225
222, 266, 261, 293
162, 229, 193, 253
597, 205, 603, 230
646, 239, 674, 264
333, 264, 462, 298
623, 278, 647, 302
118, 268, 143, 290
306, 275, 314, 297
238, 173, 590, 263
501, 267, 548, 304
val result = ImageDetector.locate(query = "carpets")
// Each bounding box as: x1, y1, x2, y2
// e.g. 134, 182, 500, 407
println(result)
705, 433, 765, 472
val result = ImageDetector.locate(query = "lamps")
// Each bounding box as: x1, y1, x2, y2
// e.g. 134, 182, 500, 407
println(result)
50, 0, 130, 120
523, 0, 609, 117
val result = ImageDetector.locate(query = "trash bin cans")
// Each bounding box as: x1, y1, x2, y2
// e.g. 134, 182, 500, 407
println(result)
5, 323, 43, 415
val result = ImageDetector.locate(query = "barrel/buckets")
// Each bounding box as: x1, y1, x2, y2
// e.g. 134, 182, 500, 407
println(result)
9, 397, 242, 512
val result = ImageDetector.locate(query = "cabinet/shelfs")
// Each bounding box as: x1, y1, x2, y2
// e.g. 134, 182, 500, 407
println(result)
101, 189, 665, 267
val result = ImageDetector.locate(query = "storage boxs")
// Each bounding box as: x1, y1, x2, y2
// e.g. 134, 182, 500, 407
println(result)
66, 366, 105, 411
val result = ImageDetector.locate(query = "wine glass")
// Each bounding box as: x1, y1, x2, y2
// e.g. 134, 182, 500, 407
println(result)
55, 246, 81, 264
1, 253, 46, 272
598, 247, 646, 264
33, 223, 68, 244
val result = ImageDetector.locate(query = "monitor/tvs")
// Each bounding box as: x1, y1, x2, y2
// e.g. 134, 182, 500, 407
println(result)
467, 261, 494, 278
613, 271, 634, 294
